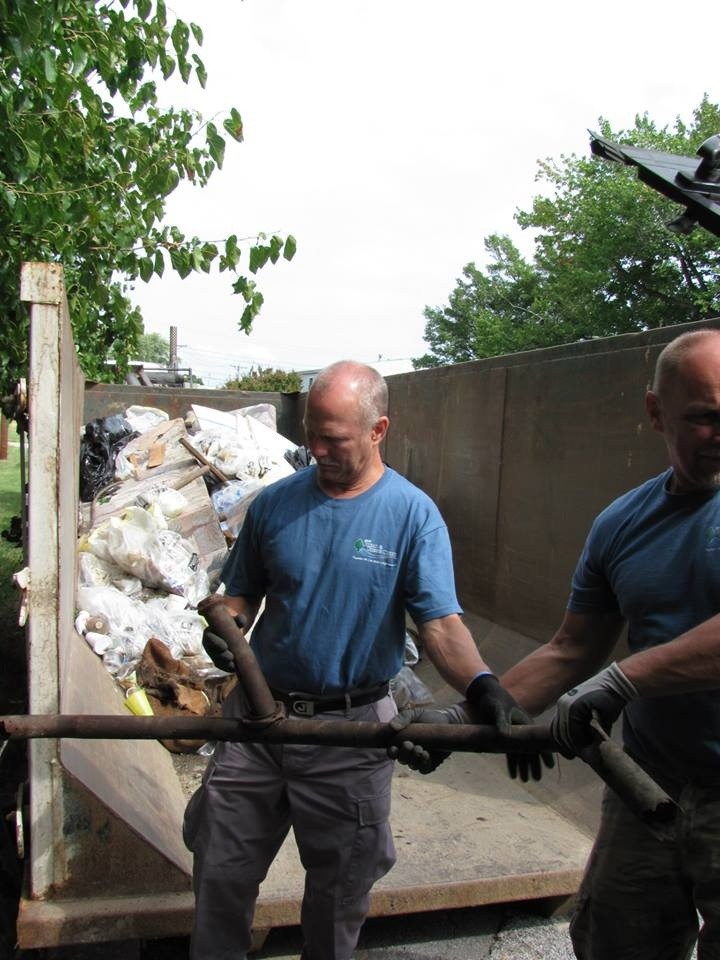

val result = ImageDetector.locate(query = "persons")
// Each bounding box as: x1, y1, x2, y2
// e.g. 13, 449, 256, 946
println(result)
388, 327, 720, 960
181, 362, 555, 960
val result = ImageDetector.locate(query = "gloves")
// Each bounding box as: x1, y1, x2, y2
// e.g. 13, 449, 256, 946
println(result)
202, 614, 247, 673
548, 660, 639, 761
387, 703, 471, 775
464, 671, 555, 783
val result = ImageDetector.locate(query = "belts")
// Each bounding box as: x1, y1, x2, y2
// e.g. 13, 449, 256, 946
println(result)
270, 682, 390, 718
